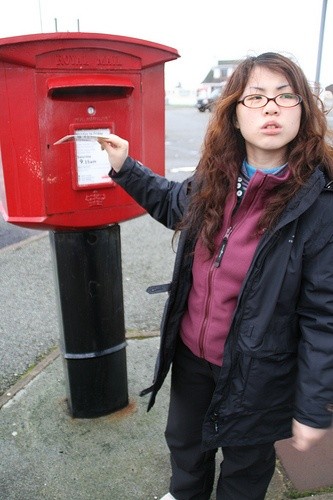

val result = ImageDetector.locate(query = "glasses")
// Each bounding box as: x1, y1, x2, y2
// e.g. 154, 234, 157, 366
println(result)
236, 93, 302, 108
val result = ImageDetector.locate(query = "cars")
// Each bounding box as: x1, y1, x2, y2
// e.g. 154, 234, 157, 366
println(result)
194, 64, 237, 114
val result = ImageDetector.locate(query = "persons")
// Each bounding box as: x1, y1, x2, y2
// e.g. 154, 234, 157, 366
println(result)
98, 53, 333, 500
